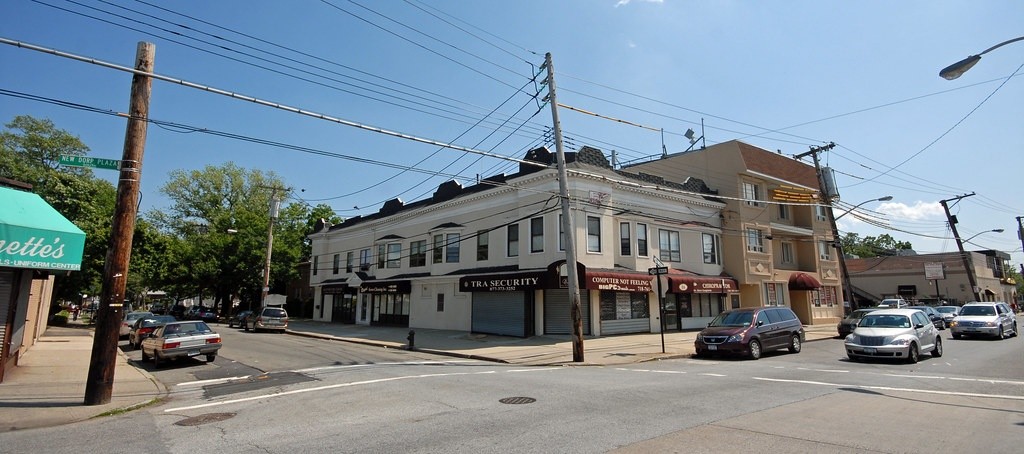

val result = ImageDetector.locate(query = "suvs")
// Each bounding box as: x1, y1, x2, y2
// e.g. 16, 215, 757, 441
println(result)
253, 306, 289, 334
694, 304, 805, 360
881, 298, 909, 309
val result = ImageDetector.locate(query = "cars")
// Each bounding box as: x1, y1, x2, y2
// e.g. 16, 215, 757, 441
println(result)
140, 319, 222, 370
147, 303, 220, 324
844, 308, 943, 364
242, 310, 260, 332
87, 307, 96, 313
128, 313, 183, 350
937, 305, 962, 328
118, 312, 155, 339
903, 305, 947, 330
949, 300, 1018, 340
228, 310, 253, 329
837, 307, 886, 340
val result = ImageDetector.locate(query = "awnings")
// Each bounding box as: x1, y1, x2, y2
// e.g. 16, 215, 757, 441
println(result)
788, 272, 820, 290
458, 271, 560, 292
0, 187, 87, 275
359, 279, 412, 295
585, 271, 738, 294
321, 283, 348, 295
985, 289, 995, 295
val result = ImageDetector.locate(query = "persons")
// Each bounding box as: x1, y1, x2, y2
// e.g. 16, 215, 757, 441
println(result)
73, 308, 78, 321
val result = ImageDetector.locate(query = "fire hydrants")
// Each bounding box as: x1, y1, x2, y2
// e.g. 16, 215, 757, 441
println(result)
407, 328, 416, 351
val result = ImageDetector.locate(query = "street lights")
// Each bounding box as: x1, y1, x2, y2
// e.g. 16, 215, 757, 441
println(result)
832, 195, 893, 313
959, 228, 1005, 302
